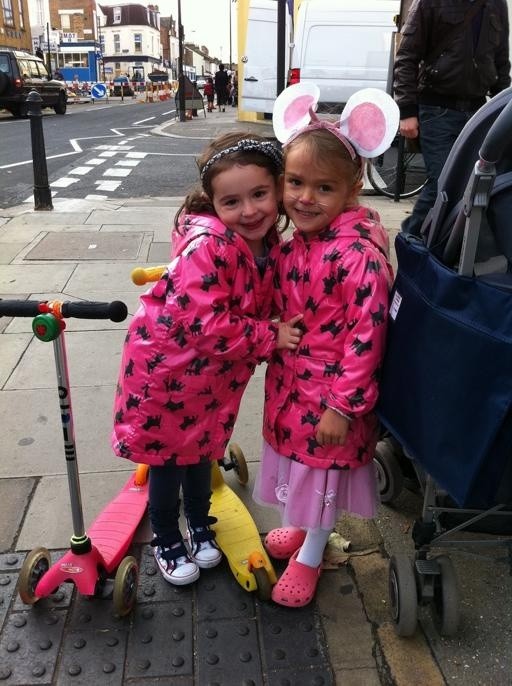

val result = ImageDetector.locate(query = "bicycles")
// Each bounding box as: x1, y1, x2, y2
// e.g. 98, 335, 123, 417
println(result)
368, 124, 437, 203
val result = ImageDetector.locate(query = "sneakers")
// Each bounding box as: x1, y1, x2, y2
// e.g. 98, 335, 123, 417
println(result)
152, 531, 200, 586
185, 516, 222, 568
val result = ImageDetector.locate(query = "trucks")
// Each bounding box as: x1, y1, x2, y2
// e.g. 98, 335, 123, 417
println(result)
194, 73, 216, 90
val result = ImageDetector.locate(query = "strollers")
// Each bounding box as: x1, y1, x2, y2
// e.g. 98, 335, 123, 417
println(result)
369, 79, 511, 642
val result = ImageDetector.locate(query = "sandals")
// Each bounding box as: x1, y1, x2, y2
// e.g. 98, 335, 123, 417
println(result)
264, 526, 305, 559
271, 548, 323, 607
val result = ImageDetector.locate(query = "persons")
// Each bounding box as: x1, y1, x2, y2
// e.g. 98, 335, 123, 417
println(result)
251, 121, 395, 607
392, 0, 512, 238
203, 64, 238, 113
111, 134, 304, 584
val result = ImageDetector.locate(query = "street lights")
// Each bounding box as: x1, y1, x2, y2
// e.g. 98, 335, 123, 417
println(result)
151, 59, 163, 98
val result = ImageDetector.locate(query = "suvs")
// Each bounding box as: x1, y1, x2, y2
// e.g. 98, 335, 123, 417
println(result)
0, 47, 69, 120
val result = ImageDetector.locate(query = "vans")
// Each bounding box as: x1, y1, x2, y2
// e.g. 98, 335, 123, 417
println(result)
238, 1, 404, 131
